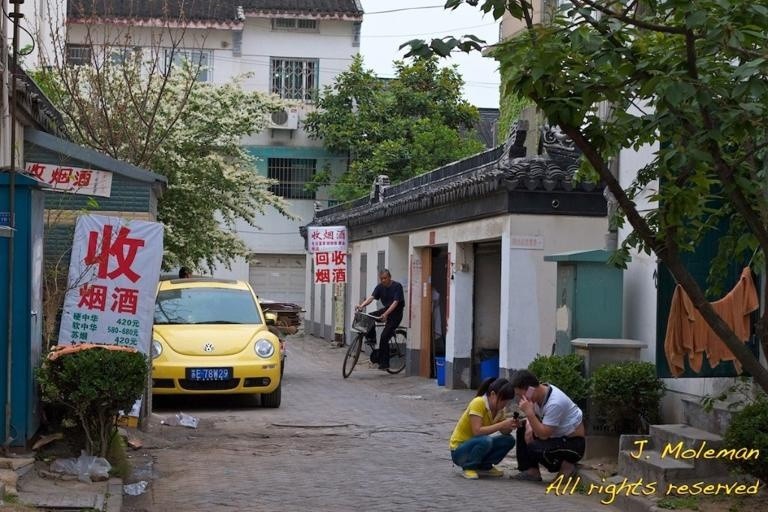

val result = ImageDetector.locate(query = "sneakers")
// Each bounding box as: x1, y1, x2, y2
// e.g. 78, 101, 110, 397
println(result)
365, 341, 376, 344
462, 467, 504, 479
378, 364, 389, 369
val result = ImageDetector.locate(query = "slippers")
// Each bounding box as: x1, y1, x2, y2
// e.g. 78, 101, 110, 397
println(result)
510, 471, 542, 481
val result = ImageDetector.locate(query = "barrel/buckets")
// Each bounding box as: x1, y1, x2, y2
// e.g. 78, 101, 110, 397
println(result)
480, 356, 499, 380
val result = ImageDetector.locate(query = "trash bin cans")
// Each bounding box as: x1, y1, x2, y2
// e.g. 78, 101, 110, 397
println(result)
478, 348, 499, 382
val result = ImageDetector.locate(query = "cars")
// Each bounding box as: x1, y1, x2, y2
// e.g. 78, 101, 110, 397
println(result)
149, 276, 282, 407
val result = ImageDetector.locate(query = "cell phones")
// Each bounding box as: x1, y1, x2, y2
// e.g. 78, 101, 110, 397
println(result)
524, 386, 535, 403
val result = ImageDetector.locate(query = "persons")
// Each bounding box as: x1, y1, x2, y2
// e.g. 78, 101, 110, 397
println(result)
179, 267, 193, 278
447, 376, 522, 478
509, 369, 586, 484
355, 269, 405, 370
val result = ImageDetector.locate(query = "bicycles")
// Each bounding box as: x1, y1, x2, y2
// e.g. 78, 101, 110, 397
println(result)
342, 308, 407, 379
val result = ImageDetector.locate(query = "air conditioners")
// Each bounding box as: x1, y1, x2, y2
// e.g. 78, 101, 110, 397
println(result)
266, 106, 298, 131
